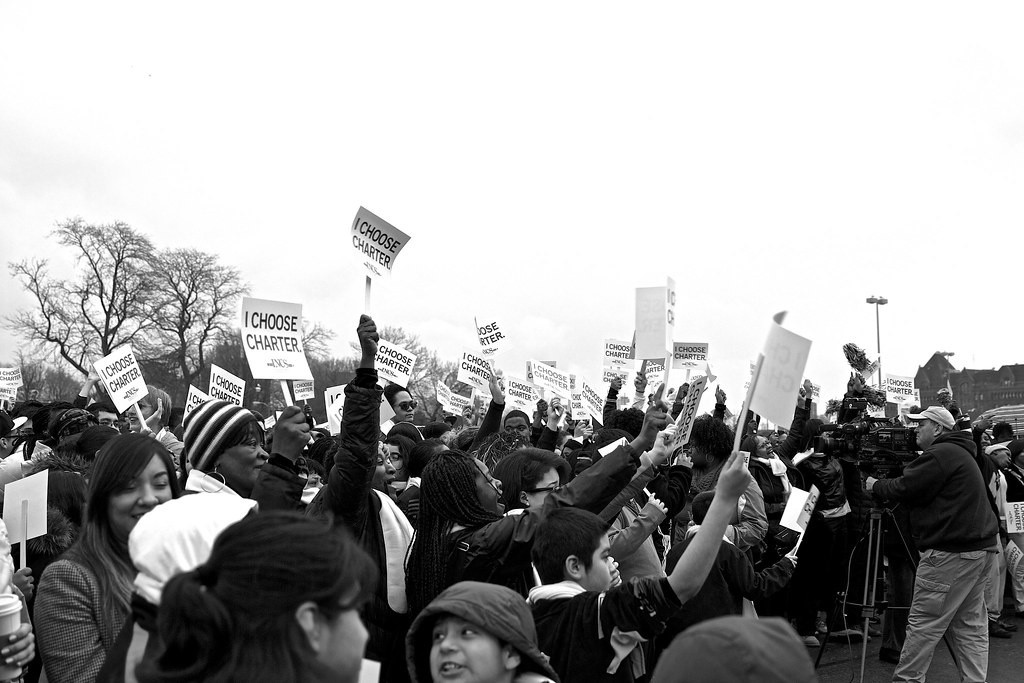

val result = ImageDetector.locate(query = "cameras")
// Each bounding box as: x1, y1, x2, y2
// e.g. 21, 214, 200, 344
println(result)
304, 416, 314, 430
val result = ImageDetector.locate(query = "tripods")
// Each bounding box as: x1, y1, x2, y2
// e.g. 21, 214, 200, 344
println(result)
815, 500, 956, 683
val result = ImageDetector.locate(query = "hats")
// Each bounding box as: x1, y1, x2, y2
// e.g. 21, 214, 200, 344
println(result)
986, 444, 1009, 456
183, 399, 257, 471
564, 436, 583, 450
0, 409, 28, 439
904, 405, 956, 430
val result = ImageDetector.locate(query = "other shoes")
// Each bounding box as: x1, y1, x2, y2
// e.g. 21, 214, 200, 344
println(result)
816, 618, 828, 633
853, 625, 873, 642
989, 622, 1011, 638
879, 647, 900, 664
830, 629, 863, 642
998, 619, 1018, 632
862, 622, 882, 636
805, 636, 820, 647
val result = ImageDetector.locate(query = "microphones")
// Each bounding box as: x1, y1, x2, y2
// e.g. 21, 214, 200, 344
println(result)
819, 424, 837, 431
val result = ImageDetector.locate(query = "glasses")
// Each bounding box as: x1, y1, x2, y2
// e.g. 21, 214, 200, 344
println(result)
390, 453, 403, 461
756, 440, 771, 451
137, 403, 153, 410
527, 486, 559, 494
101, 421, 122, 429
392, 401, 417, 410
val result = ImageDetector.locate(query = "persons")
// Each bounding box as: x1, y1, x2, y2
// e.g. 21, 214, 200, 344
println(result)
0, 316, 1024, 683
864, 406, 999, 683
